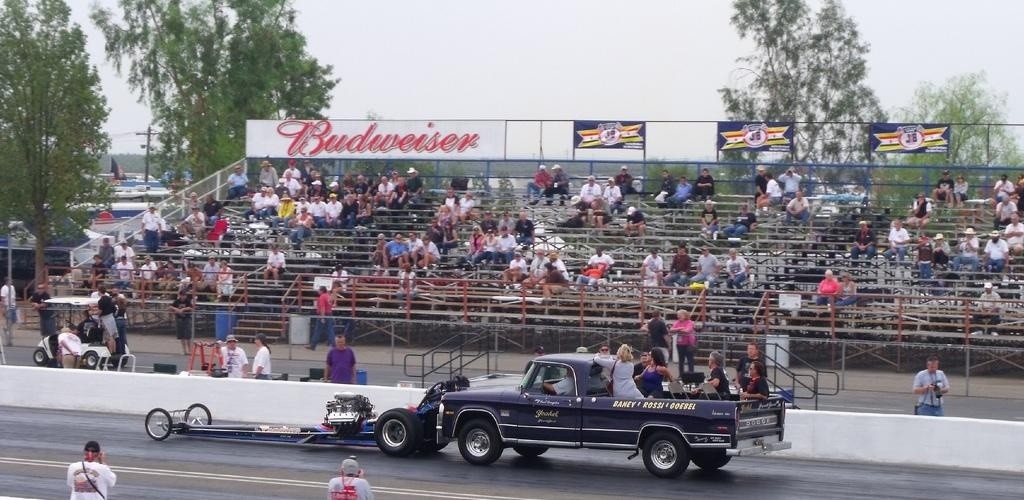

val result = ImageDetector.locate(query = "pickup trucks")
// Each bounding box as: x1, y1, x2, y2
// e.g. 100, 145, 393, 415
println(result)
439, 351, 794, 477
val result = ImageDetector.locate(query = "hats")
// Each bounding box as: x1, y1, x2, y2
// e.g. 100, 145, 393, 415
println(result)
224, 335, 238, 344
341, 459, 359, 475
407, 168, 417, 173
119, 239, 127, 244
540, 165, 627, 205
933, 233, 943, 240
988, 231, 1000, 237
964, 227, 976, 235
984, 282, 993, 289
85, 441, 100, 452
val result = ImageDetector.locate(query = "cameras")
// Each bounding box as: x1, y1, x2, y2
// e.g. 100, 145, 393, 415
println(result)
931, 380, 943, 398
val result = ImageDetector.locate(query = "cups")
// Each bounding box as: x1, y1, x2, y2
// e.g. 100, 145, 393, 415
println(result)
617, 270, 623, 278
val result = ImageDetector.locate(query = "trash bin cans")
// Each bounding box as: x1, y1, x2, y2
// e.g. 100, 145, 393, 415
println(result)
356, 369, 367, 385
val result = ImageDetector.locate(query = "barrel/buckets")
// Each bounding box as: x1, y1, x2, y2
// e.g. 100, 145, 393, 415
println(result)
355, 369, 366, 384
154, 364, 176, 373
15, 307, 28, 324
309, 367, 325, 380
918, 260, 937, 279
672, 333, 686, 364
770, 387, 793, 403
37, 307, 61, 337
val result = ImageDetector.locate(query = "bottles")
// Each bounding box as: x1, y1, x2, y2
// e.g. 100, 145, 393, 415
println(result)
683, 382, 696, 393
935, 380, 943, 400
763, 205, 768, 212
739, 386, 743, 394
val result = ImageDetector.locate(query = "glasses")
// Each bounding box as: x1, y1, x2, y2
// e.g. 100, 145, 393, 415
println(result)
601, 350, 608, 351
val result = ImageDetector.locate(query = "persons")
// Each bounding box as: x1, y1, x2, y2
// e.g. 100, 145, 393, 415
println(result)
0, 276, 18, 331
328, 458, 373, 500
67, 441, 116, 500
30, 154, 1024, 418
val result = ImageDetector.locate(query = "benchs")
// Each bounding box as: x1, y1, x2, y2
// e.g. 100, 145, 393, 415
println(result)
20, 178, 1019, 350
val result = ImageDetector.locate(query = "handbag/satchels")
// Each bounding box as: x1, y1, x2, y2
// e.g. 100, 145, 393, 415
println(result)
607, 379, 613, 394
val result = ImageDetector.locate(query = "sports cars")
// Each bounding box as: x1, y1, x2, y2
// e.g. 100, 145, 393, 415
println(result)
147, 374, 471, 459
